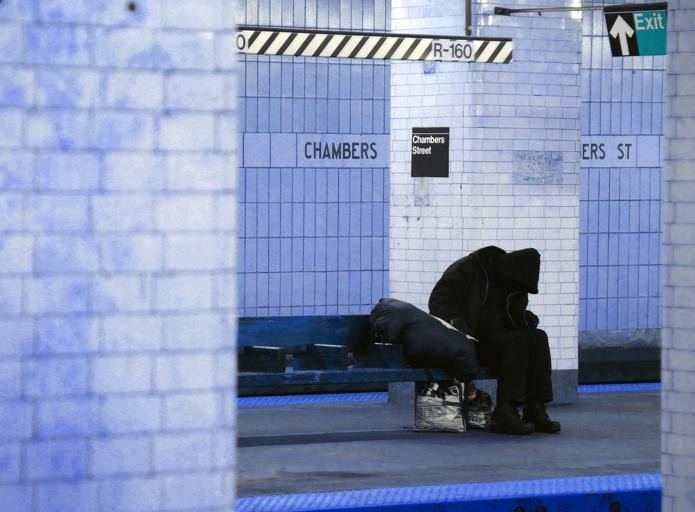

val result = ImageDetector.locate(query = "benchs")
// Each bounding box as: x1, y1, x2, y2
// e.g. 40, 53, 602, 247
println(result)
240, 309, 523, 395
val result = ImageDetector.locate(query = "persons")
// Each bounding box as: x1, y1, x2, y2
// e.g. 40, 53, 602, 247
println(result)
428, 245, 563, 434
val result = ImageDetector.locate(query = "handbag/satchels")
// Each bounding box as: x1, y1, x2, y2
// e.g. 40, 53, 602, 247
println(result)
415, 374, 466, 433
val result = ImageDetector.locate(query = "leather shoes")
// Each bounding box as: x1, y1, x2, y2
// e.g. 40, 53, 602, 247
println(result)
489, 407, 535, 434
523, 404, 560, 432
